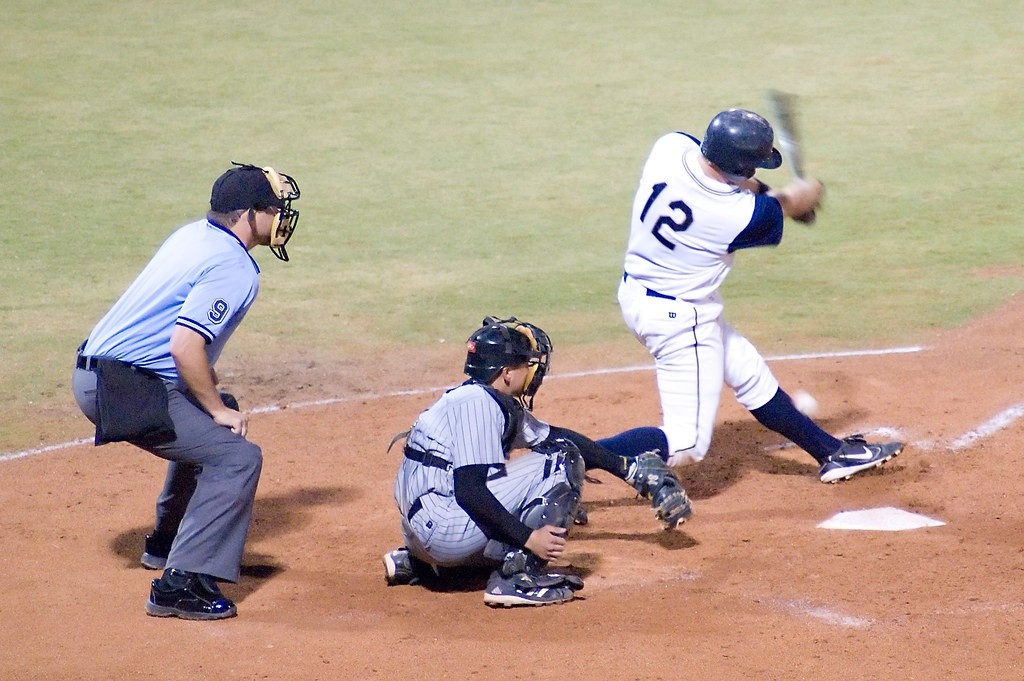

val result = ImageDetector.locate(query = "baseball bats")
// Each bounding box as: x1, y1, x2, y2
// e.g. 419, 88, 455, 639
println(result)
767, 86, 818, 223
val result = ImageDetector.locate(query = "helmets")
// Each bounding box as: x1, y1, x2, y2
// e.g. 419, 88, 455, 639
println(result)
464, 324, 531, 383
701, 108, 783, 179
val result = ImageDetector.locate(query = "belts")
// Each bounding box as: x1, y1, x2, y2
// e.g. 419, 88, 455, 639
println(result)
407, 491, 439, 523
624, 271, 676, 300
76, 355, 131, 372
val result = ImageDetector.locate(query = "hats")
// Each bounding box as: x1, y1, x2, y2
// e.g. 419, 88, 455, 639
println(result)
209, 167, 285, 213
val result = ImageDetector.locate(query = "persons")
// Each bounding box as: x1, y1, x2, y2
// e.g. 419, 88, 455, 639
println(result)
72, 162, 301, 620
584, 108, 906, 485
382, 316, 693, 607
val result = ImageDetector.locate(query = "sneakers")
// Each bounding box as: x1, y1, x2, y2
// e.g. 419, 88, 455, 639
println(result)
383, 544, 416, 582
573, 505, 589, 525
483, 569, 574, 607
146, 572, 237, 620
140, 533, 174, 570
819, 434, 903, 483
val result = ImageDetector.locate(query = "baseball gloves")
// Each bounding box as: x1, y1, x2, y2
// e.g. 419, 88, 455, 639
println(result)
631, 451, 692, 529
